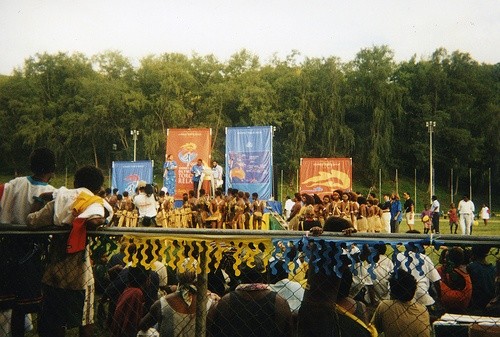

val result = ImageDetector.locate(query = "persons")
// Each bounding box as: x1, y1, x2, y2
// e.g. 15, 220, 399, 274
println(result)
164, 154, 178, 196
481, 203, 490, 226
403, 191, 415, 230
0, 148, 58, 337
421, 195, 442, 234
96, 182, 265, 230
458, 195, 475, 234
285, 189, 402, 232
27, 167, 115, 337
91, 239, 500, 336
212, 161, 223, 188
191, 159, 205, 193
447, 203, 458, 234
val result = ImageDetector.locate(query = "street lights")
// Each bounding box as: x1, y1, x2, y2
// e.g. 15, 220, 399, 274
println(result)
130, 130, 141, 162
425, 120, 436, 207
